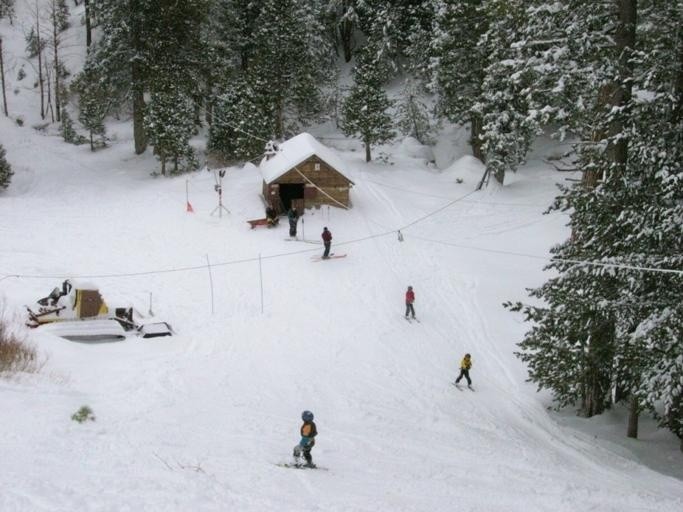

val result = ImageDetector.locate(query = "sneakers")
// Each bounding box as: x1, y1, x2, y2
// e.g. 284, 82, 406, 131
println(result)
289, 463, 301, 469
303, 463, 314, 467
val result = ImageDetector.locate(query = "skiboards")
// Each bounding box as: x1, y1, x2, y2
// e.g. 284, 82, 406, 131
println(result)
314, 253, 346, 262
286, 239, 321, 245
402, 315, 421, 325
274, 464, 324, 471
453, 381, 475, 391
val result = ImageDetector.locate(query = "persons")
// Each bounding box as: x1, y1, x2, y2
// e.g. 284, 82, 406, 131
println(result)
404, 285, 416, 320
292, 410, 317, 469
265, 202, 279, 230
454, 353, 474, 389
321, 226, 333, 259
286, 205, 299, 239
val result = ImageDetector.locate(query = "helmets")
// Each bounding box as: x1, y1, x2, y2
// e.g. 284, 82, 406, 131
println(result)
302, 410, 314, 422
465, 354, 470, 358
